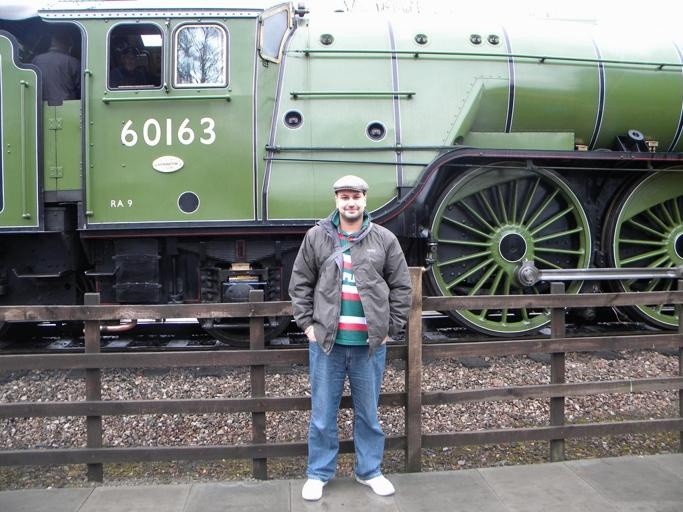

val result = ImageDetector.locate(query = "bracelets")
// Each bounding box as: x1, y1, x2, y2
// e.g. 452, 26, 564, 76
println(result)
304, 325, 312, 337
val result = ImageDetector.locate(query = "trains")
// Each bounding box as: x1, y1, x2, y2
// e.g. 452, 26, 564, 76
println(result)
1, 0, 682, 341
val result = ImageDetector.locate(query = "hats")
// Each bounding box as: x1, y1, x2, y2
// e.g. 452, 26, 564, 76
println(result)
333, 176, 368, 192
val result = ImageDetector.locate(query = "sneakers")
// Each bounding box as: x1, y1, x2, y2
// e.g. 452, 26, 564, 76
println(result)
302, 478, 328, 500
355, 473, 395, 496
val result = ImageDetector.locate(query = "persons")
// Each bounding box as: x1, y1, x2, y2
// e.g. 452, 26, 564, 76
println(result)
108, 44, 161, 87
30, 28, 81, 107
288, 173, 411, 501
